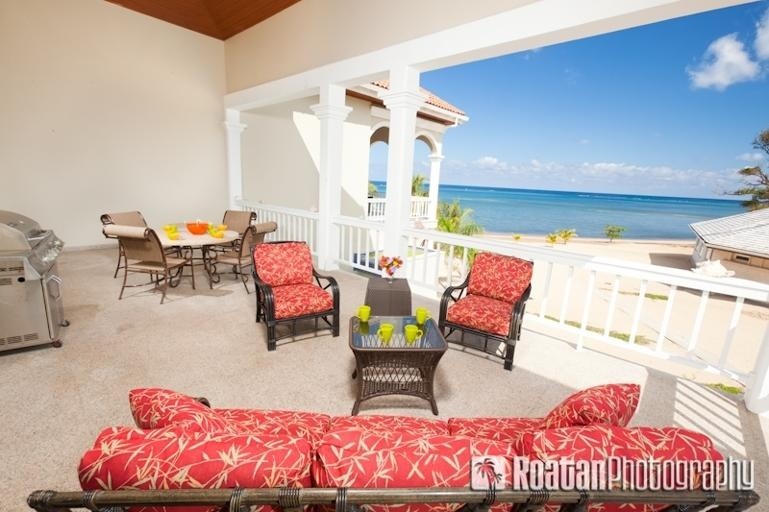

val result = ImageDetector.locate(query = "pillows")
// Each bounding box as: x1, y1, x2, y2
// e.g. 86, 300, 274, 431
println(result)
127, 388, 239, 440
535, 383, 640, 431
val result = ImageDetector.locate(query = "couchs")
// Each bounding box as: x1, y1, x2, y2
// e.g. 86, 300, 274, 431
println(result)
25, 396, 758, 512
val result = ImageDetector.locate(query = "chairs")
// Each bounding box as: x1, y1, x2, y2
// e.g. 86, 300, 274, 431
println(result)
100, 210, 276, 305
249, 240, 339, 351
438, 249, 533, 370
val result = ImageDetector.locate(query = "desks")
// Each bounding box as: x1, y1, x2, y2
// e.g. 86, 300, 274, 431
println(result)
363, 278, 411, 315
349, 315, 448, 416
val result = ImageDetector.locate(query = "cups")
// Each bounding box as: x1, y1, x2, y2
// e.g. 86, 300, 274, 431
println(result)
404, 324, 423, 343
356, 305, 371, 322
416, 307, 431, 325
377, 324, 394, 343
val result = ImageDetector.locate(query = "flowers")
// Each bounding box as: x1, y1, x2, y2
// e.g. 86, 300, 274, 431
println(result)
378, 256, 403, 275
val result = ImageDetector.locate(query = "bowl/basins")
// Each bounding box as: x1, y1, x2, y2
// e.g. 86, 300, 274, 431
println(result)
186, 222, 209, 234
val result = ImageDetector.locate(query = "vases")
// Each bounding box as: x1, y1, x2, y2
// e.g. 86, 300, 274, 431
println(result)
387, 275, 393, 284
